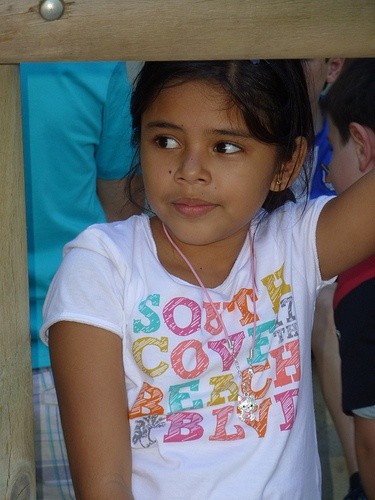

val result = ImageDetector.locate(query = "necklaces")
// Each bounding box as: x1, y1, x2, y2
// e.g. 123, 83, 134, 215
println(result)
161, 222, 255, 422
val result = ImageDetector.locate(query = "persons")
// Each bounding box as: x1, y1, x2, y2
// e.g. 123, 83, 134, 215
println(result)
21, 63, 145, 500
39, 58, 375, 500
288, 62, 366, 500
320, 60, 375, 500
